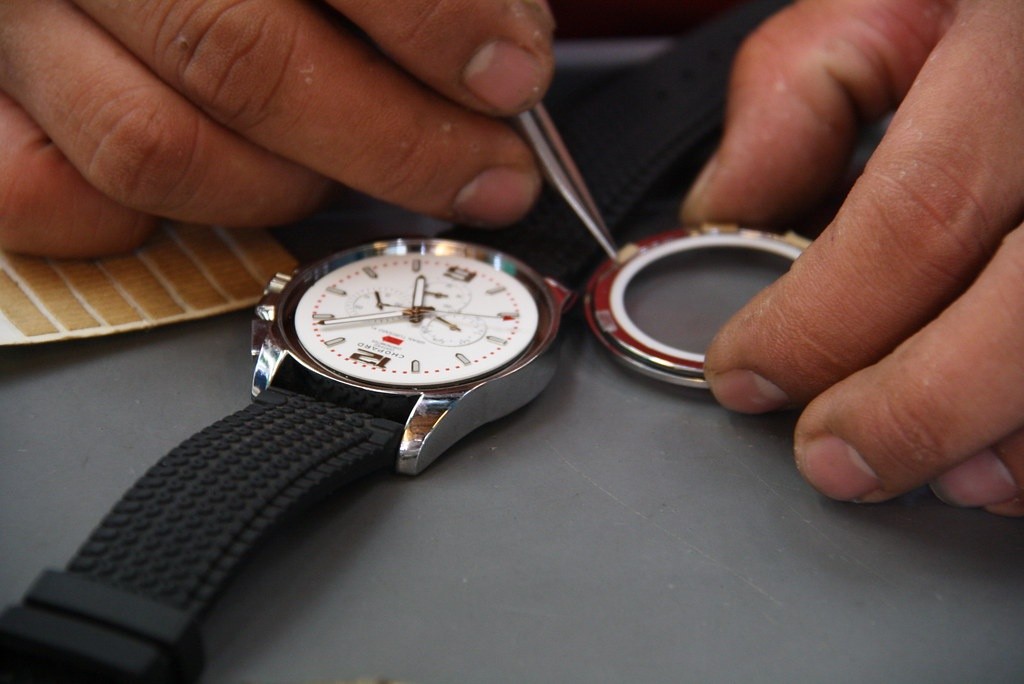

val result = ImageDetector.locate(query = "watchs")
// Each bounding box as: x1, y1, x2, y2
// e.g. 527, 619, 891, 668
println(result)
0, 0, 789, 684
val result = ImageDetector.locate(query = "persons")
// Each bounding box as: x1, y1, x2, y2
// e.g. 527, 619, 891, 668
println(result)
0, 0, 1024, 518
